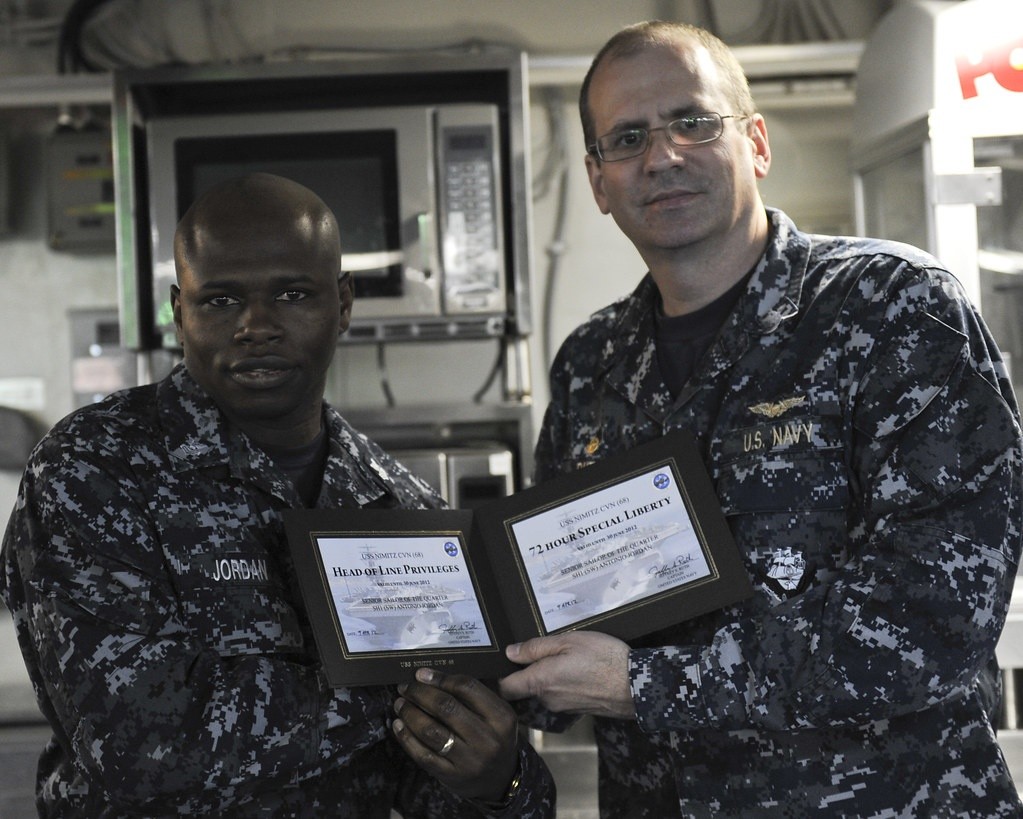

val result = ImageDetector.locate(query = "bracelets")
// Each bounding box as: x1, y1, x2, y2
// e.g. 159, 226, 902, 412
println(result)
481, 769, 522, 809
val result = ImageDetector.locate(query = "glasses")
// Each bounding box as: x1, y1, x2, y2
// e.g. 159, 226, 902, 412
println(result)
587, 111, 751, 163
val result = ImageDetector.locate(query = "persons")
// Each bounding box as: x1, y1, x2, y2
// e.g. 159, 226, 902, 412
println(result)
498, 19, 1022, 819
0, 172, 560, 819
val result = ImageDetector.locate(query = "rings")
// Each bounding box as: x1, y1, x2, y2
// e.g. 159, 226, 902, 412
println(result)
439, 733, 455, 758
